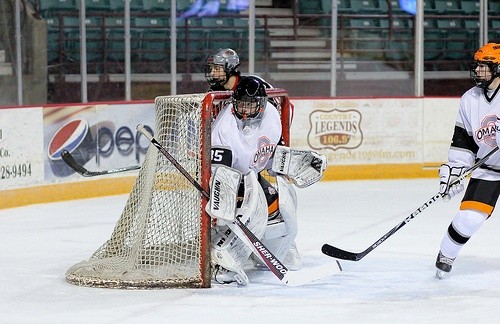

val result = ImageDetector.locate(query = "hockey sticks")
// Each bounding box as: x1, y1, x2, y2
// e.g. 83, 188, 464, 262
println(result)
136, 122, 345, 287
61, 149, 142, 177
321, 147, 500, 262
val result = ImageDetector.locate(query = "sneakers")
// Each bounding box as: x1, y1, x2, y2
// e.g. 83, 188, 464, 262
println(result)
436, 251, 454, 279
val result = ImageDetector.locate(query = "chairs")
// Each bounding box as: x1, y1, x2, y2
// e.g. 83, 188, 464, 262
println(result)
34, 0, 500, 73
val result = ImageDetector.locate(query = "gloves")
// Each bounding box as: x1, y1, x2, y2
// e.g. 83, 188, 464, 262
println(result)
439, 164, 465, 203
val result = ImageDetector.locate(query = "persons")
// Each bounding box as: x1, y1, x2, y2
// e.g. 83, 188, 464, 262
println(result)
436, 43, 500, 279
202, 48, 324, 285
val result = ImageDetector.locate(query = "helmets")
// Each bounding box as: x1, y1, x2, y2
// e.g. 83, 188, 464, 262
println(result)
232, 76, 267, 122
469, 43, 500, 88
204, 48, 240, 87
